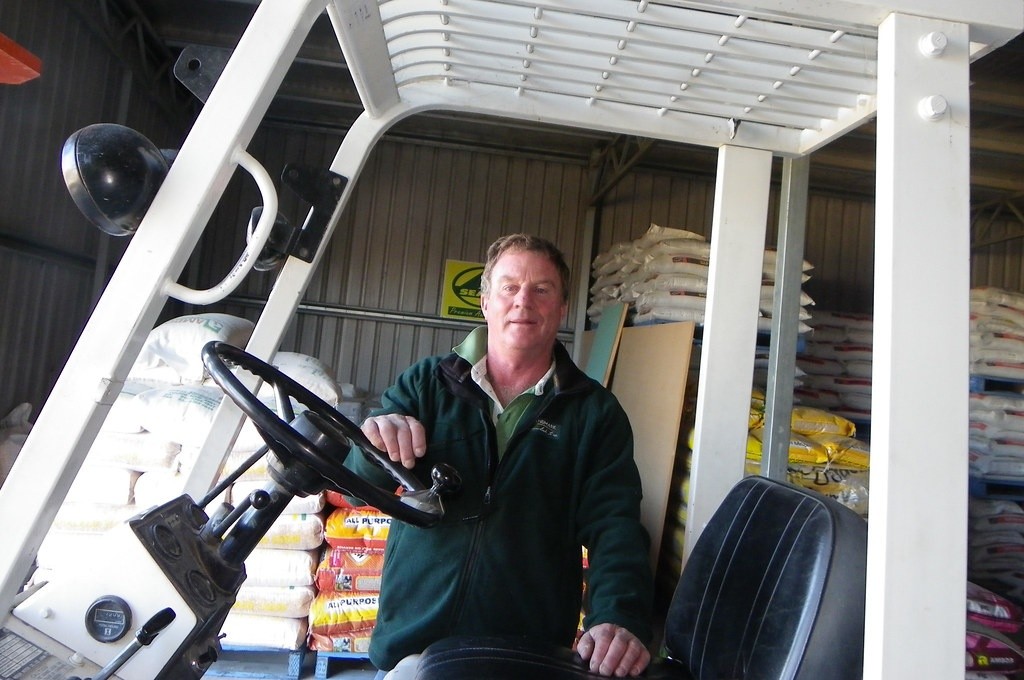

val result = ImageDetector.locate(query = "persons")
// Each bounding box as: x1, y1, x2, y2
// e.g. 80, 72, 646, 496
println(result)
340, 233, 658, 680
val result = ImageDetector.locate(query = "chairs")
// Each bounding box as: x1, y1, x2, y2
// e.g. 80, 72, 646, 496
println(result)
413, 475, 867, 680
